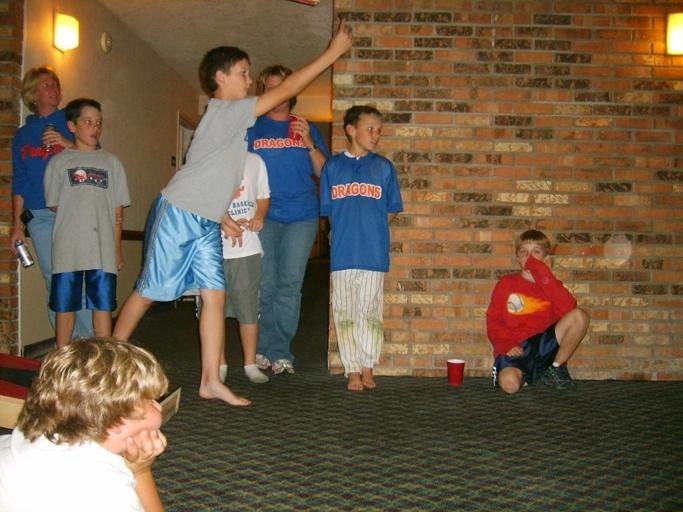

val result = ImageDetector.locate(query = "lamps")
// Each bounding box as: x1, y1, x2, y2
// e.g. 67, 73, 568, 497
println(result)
51, 8, 79, 54
665, 12, 683, 56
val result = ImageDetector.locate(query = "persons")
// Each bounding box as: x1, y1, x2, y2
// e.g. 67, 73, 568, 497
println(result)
195, 152, 271, 384
0, 337, 169, 512
42, 98, 132, 350
319, 104, 404, 392
247, 64, 332, 376
11, 67, 96, 346
486, 229, 590, 395
112, 18, 354, 408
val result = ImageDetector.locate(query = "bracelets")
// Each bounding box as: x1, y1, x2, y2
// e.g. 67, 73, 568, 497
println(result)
307, 144, 316, 152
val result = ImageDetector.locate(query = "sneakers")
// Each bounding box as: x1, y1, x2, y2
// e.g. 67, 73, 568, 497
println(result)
542, 363, 573, 390
272, 358, 297, 377
253, 353, 272, 370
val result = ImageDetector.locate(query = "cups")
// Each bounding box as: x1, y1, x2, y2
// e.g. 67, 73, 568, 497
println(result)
446, 358, 466, 387
288, 114, 302, 141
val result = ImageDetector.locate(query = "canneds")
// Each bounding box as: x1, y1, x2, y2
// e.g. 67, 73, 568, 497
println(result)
14, 241, 34, 268
